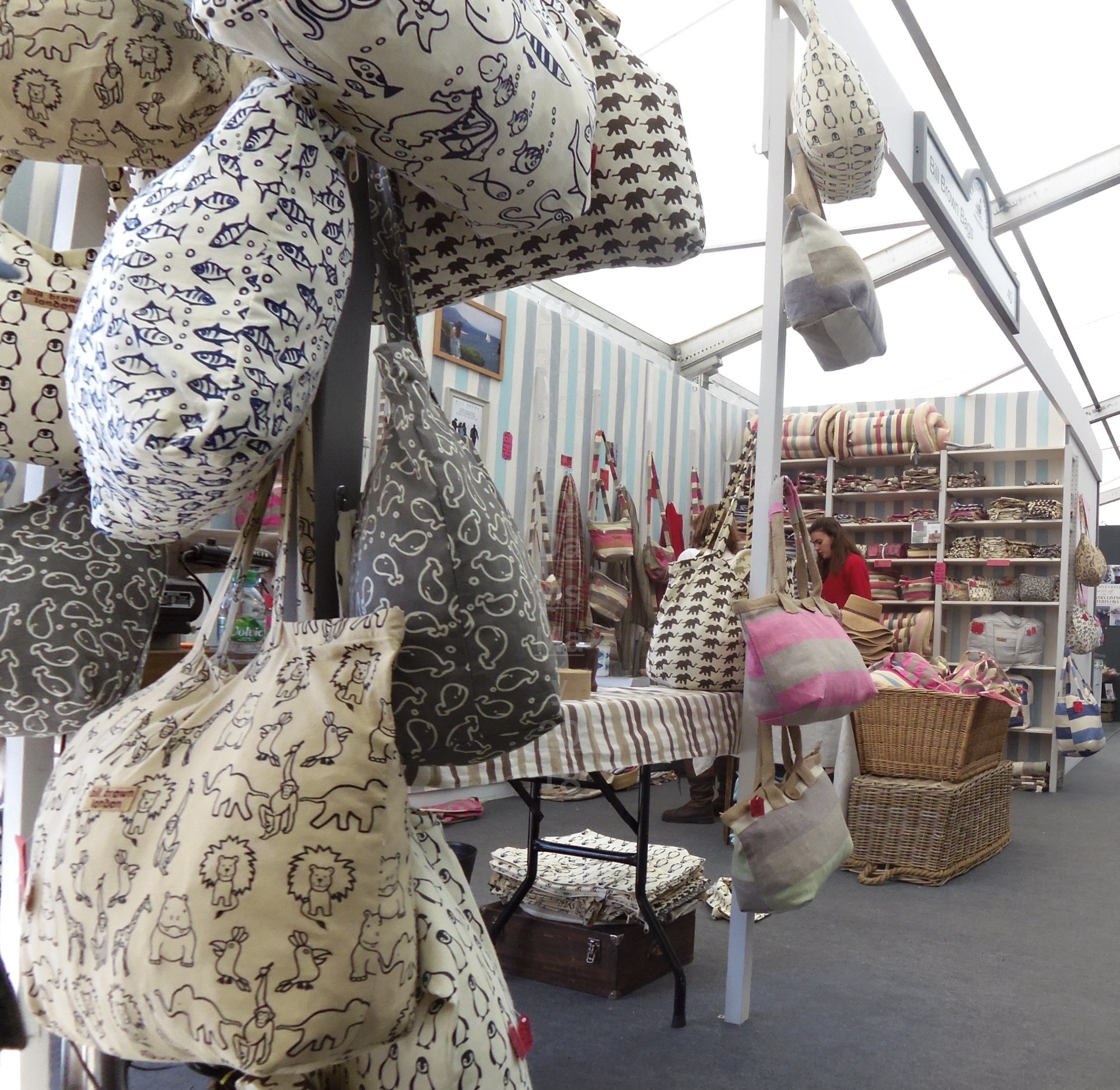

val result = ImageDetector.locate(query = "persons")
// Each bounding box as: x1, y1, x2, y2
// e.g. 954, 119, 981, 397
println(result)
662, 504, 739, 823
809, 517, 872, 608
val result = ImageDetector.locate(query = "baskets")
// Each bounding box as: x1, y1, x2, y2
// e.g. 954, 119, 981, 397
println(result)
851, 686, 1014, 783
611, 765, 640, 790
840, 759, 1014, 886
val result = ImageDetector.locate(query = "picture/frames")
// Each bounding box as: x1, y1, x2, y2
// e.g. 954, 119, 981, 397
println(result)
433, 300, 507, 382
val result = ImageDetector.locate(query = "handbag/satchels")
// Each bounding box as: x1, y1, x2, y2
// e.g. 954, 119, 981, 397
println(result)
1001, 673, 1036, 730
1067, 579, 1104, 654
1055, 645, 1106, 757
646, 431, 795, 691
867, 570, 1060, 604
790, 1, 886, 203
867, 646, 1024, 706
965, 611, 1044, 673
1076, 496, 1107, 587
732, 475, 879, 726
719, 715, 853, 915
782, 133, 886, 371
486, 828, 710, 934
419, 796, 483, 824
0, 0, 708, 1090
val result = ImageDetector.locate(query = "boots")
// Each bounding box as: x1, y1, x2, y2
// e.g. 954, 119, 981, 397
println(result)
662, 758, 715, 824
713, 755, 739, 817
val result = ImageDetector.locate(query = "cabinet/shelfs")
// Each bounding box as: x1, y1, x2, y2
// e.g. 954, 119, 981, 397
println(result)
726, 444, 1079, 792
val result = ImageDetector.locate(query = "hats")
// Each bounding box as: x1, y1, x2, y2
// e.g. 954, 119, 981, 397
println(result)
838, 594, 896, 666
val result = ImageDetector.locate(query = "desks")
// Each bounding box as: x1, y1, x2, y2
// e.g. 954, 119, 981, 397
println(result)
400, 684, 739, 1030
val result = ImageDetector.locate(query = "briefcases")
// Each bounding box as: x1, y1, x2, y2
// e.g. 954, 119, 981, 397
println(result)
478, 900, 695, 999
